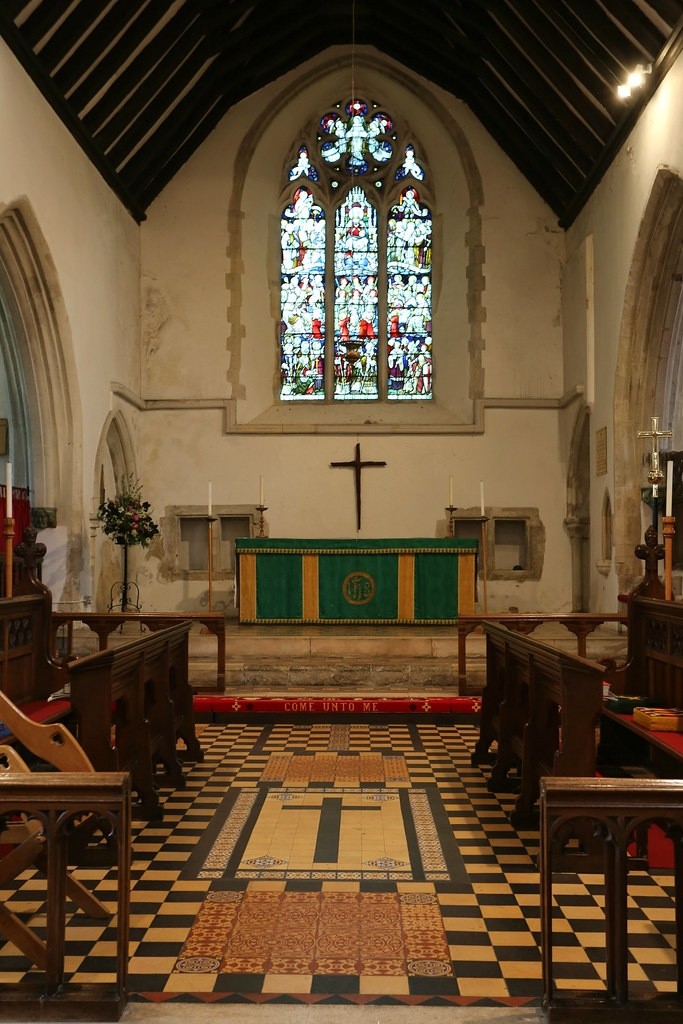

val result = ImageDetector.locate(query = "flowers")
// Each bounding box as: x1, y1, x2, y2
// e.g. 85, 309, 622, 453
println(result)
92, 470, 160, 549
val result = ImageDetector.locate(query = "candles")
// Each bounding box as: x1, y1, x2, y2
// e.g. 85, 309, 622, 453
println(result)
480, 480, 484, 516
208, 481, 212, 516
666, 460, 673, 516
6, 461, 12, 519
449, 475, 453, 505
260, 476, 264, 505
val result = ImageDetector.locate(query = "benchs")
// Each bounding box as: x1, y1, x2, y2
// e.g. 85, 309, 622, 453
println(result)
603, 695, 683, 774
0, 693, 75, 753
